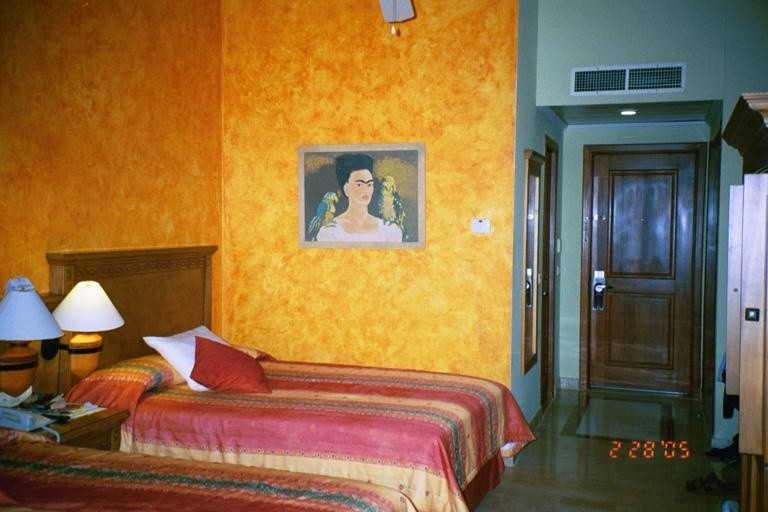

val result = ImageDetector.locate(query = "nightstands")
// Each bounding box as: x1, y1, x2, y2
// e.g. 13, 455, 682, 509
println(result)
31, 405, 131, 452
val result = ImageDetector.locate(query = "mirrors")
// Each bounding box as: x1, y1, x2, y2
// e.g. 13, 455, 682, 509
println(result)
521, 148, 546, 375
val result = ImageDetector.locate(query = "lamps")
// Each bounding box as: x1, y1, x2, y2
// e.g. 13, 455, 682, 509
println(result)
0, 277, 64, 397
41, 280, 126, 379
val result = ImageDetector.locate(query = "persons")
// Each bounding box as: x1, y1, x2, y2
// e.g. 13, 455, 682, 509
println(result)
314, 153, 403, 243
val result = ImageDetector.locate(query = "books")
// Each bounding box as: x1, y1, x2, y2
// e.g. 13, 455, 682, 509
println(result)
42, 403, 107, 423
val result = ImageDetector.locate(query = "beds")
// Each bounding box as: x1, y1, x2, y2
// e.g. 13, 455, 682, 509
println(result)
46, 246, 537, 512
0, 442, 416, 512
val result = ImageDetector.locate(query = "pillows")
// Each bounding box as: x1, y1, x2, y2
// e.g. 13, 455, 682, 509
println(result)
65, 324, 272, 409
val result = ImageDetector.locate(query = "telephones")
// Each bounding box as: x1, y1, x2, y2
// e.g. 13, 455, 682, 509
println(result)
0, 405, 56, 432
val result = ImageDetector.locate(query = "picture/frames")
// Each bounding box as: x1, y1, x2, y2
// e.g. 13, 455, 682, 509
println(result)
299, 144, 426, 249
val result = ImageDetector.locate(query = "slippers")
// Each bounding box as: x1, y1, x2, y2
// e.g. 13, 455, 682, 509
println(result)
688, 473, 738, 495
